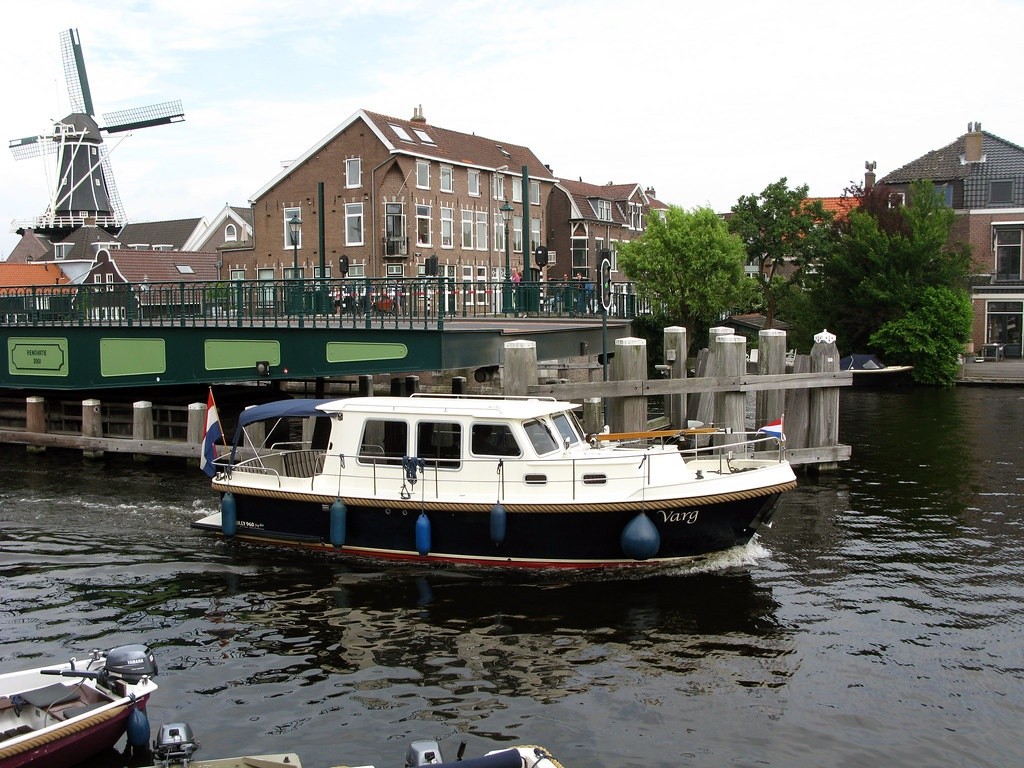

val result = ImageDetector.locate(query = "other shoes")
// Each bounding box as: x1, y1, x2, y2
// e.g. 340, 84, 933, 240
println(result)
523, 314, 527, 318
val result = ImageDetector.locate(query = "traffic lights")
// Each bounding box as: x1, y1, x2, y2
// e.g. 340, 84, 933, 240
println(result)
600, 258, 614, 310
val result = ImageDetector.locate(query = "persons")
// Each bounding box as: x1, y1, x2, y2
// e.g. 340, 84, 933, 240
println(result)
512, 268, 592, 313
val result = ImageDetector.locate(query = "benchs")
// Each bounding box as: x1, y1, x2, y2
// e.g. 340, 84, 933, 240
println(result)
23, 683, 108, 730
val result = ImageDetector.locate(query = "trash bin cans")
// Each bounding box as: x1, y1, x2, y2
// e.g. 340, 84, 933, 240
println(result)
291, 291, 337, 314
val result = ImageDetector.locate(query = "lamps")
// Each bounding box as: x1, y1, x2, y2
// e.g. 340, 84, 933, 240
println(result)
595, 352, 616, 366
339, 255, 349, 277
471, 364, 500, 383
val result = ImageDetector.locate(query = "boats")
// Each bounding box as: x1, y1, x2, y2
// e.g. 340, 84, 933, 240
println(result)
188, 385, 800, 569
133, 722, 304, 768
0, 642, 160, 768
689, 346, 915, 390
329, 738, 565, 768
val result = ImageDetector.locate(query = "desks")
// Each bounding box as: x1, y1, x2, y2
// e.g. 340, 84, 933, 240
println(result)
983, 343, 1005, 361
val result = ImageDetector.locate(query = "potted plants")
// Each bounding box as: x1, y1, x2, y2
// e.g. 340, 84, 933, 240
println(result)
976, 356, 984, 363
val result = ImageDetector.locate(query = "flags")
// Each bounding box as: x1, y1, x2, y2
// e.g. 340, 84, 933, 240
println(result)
200, 392, 222, 477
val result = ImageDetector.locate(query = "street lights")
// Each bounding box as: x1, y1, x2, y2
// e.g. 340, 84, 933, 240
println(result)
498, 195, 516, 313
288, 212, 303, 313
